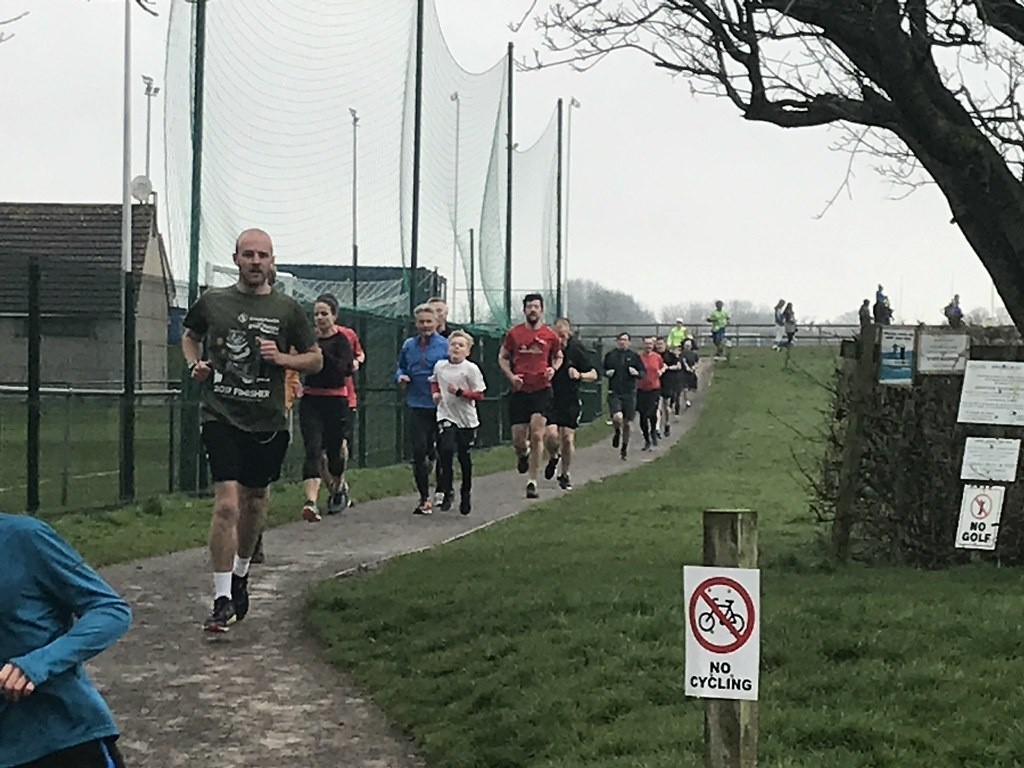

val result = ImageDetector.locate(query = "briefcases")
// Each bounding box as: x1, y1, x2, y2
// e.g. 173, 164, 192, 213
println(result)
621, 450, 627, 460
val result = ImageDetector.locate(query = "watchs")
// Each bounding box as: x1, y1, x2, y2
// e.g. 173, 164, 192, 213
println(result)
187, 362, 196, 374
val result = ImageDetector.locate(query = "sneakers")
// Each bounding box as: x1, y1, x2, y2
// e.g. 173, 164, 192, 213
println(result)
517, 448, 530, 474
526, 482, 538, 498
441, 493, 454, 511
558, 473, 572, 491
201, 595, 236, 632
413, 500, 432, 514
434, 492, 444, 505
328, 480, 348, 513
231, 572, 250, 620
302, 500, 321, 522
545, 453, 562, 480
459, 488, 471, 514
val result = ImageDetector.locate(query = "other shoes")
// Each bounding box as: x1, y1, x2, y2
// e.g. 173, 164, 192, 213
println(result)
250, 544, 264, 563
642, 399, 691, 450
613, 434, 619, 448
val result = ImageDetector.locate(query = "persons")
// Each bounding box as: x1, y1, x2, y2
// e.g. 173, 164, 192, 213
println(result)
181, 228, 323, 634
652, 335, 700, 439
499, 294, 564, 499
601, 332, 647, 461
395, 305, 451, 515
707, 300, 730, 358
774, 299, 797, 346
0, 511, 133, 768
428, 329, 486, 516
638, 337, 665, 451
296, 298, 354, 523
250, 263, 299, 564
543, 317, 598, 492
944, 294, 964, 326
426, 297, 451, 508
314, 294, 366, 507
859, 285, 895, 328
667, 318, 694, 356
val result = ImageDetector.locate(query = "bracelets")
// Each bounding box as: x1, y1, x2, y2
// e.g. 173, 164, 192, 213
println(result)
455, 388, 463, 397
